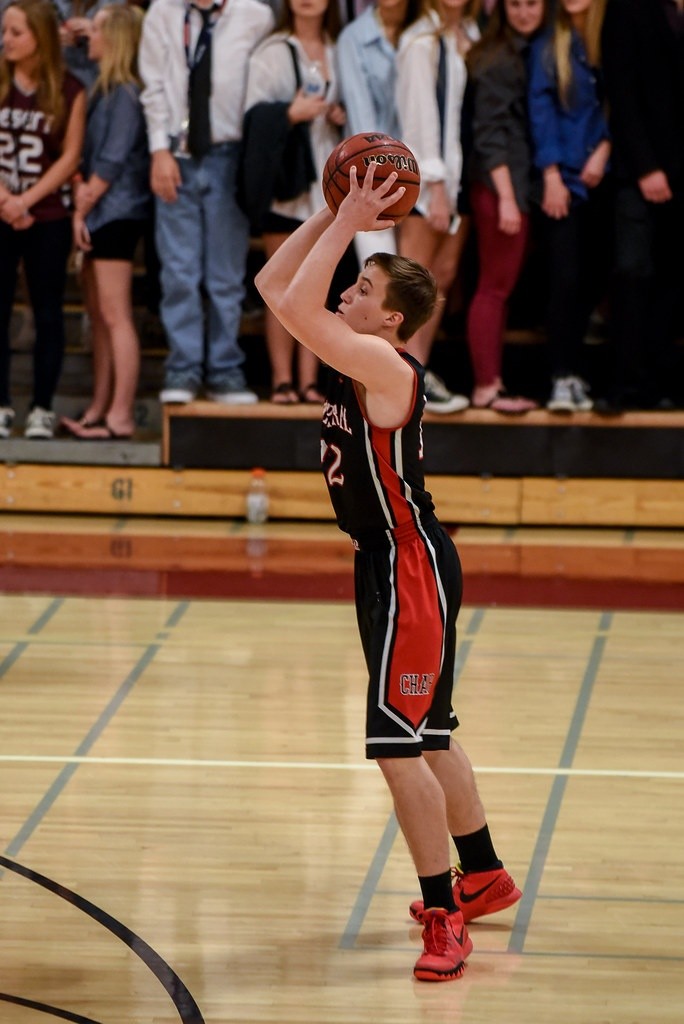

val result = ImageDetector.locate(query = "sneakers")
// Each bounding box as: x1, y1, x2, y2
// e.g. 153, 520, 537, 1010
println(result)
413, 908, 473, 981
546, 375, 594, 413
24, 405, 57, 438
410, 866, 521, 925
0, 408, 16, 438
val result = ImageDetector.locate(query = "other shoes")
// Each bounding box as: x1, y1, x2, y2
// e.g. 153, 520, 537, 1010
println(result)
421, 367, 470, 415
206, 386, 259, 404
159, 386, 197, 404
594, 382, 659, 411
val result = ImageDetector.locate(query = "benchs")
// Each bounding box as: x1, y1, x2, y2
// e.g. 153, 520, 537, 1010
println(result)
14, 262, 171, 361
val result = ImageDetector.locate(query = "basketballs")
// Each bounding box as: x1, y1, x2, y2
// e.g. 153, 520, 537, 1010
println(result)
320, 131, 423, 232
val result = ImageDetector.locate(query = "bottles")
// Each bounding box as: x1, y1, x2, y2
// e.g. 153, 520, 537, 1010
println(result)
247, 467, 269, 525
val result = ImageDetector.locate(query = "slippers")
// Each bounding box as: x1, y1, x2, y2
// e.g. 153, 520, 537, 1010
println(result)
60, 415, 136, 441
473, 386, 537, 414
268, 383, 300, 404
297, 383, 333, 404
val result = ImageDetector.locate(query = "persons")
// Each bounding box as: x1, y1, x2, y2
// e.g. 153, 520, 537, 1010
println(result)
0, 0, 684, 444
254, 160, 522, 980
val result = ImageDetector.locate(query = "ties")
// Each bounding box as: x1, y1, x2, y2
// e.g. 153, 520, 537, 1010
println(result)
186, 4, 219, 155
